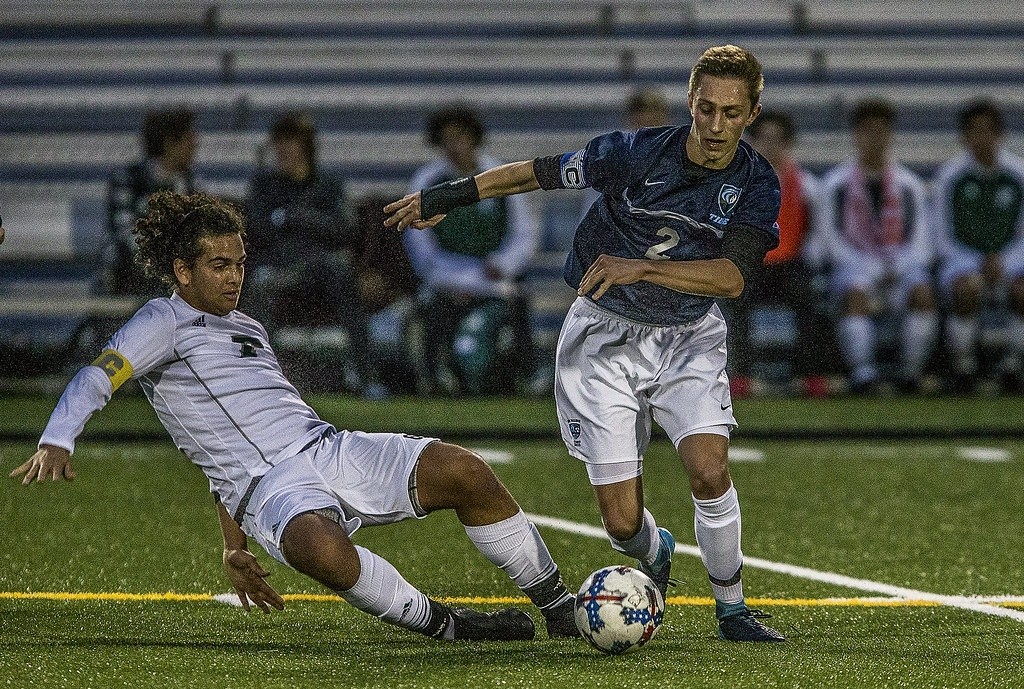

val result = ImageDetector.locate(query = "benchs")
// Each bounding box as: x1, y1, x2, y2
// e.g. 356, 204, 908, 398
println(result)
0, 0, 1024, 370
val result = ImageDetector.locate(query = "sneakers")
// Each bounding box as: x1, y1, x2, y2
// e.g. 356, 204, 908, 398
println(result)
638, 527, 684, 603
452, 606, 536, 642
545, 602, 579, 636
719, 608, 788, 643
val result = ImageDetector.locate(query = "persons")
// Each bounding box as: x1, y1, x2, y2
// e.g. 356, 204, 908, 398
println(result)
350, 197, 460, 400
243, 112, 393, 401
10, 196, 578, 639
812, 99, 937, 400
102, 108, 199, 295
724, 112, 835, 400
929, 100, 1024, 397
399, 105, 538, 394
384, 43, 781, 643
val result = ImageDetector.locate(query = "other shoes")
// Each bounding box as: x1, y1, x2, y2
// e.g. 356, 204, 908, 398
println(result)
941, 376, 974, 399
1003, 372, 1024, 397
895, 378, 922, 400
849, 380, 877, 398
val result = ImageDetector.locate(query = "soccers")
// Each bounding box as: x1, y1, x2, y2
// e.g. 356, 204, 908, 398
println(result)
574, 565, 664, 654
